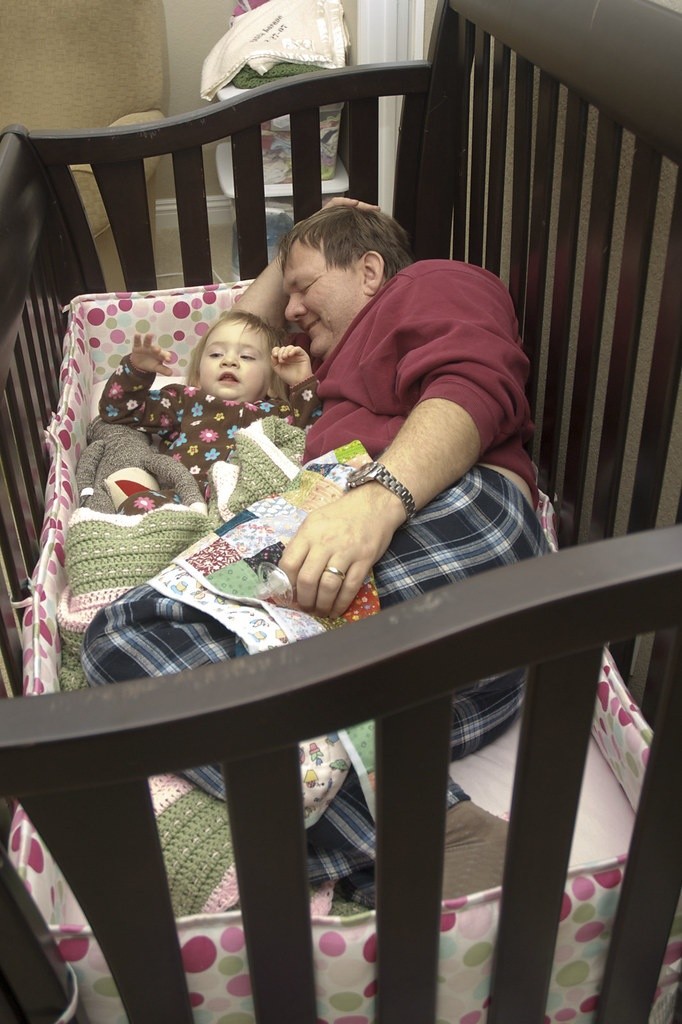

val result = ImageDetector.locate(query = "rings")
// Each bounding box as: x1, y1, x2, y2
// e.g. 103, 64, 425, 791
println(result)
324, 567, 343, 579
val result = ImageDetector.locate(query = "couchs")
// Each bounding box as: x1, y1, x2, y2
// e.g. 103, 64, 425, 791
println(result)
0, 0, 169, 297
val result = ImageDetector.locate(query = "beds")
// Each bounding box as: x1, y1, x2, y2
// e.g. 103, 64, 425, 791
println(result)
0, 0, 682, 1024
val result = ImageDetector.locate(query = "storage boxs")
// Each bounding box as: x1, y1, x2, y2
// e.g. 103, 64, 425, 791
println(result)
217, 80, 345, 184
215, 142, 350, 274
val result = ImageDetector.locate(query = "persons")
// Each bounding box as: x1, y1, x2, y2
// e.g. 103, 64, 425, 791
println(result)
82, 202, 554, 920
97, 309, 316, 516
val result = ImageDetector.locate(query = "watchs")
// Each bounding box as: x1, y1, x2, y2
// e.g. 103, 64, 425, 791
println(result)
342, 461, 418, 521
